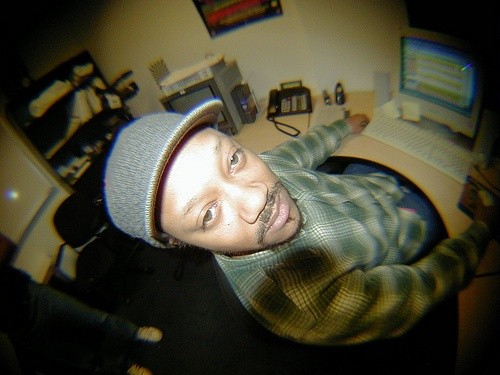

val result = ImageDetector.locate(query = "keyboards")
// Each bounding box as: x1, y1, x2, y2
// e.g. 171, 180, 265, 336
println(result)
362, 113, 473, 183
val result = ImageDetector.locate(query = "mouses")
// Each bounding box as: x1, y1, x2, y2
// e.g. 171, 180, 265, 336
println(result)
479, 190, 494, 207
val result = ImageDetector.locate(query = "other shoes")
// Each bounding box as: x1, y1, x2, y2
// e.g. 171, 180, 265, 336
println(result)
137, 326, 162, 342
127, 364, 151, 375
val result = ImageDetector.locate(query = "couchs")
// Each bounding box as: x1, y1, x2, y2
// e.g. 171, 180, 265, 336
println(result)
210, 254, 461, 375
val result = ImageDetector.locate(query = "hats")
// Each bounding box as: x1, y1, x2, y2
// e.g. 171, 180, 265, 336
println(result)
105, 98, 224, 246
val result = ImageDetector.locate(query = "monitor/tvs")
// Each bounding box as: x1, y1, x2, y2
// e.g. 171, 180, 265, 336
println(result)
390, 25, 482, 137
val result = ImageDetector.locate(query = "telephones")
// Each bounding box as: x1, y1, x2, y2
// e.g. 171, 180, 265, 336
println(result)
262, 79, 315, 138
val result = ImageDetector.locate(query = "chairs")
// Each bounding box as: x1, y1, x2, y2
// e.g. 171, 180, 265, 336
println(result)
52, 194, 141, 313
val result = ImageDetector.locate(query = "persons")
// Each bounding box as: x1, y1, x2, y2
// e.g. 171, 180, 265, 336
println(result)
103, 114, 498, 346
1, 258, 162, 375
